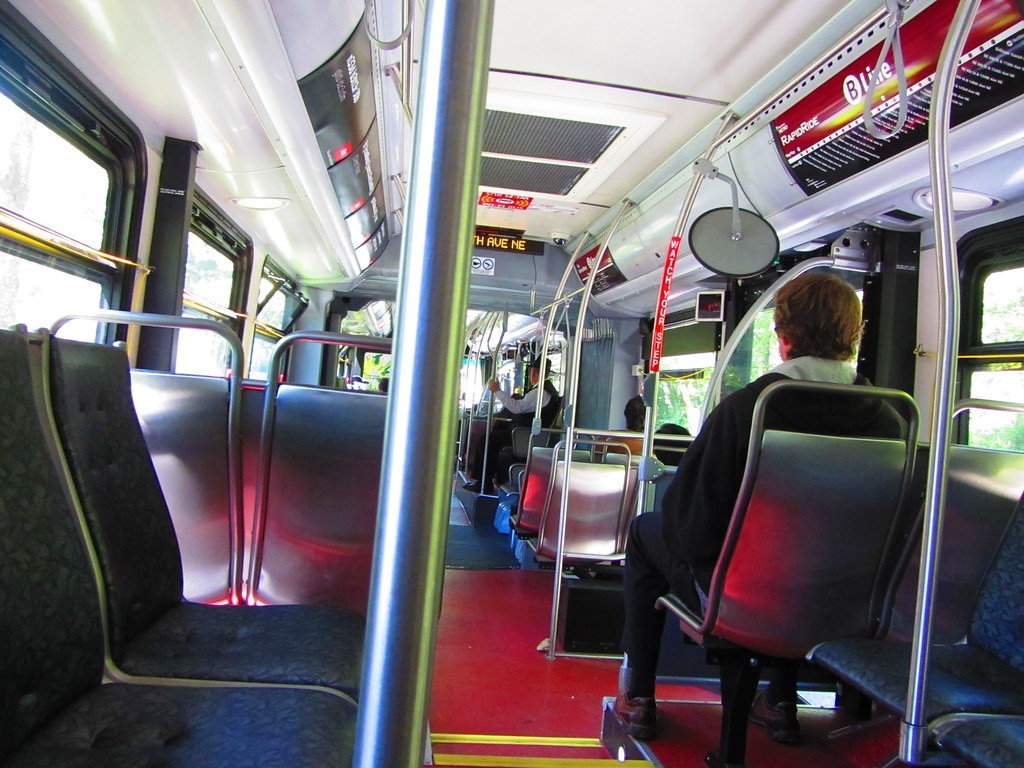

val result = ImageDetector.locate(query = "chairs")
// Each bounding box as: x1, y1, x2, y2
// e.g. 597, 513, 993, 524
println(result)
0, 309, 1022, 763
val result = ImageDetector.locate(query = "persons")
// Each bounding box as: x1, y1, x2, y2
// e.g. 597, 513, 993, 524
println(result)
654, 423, 690, 466
607, 395, 646, 453
617, 273, 905, 743
463, 356, 560, 492
377, 378, 388, 392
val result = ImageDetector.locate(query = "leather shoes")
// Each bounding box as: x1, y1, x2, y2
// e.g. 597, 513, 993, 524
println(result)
747, 693, 801, 745
614, 689, 658, 739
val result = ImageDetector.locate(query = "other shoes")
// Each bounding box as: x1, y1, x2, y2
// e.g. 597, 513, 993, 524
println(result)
462, 481, 495, 493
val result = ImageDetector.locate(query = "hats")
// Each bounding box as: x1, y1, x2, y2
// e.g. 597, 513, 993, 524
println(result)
526, 354, 556, 373
